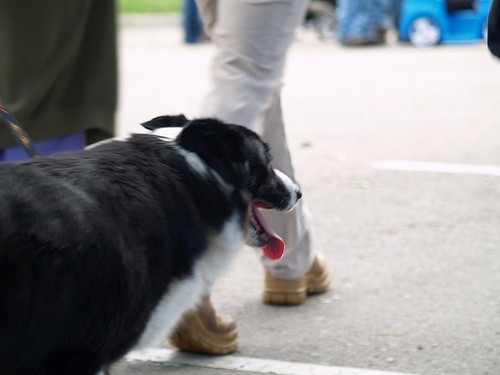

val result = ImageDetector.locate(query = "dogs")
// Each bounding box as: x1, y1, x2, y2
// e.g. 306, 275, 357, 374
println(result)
0, 118, 303, 375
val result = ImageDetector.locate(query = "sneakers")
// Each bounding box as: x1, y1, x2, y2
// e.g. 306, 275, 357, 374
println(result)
169, 304, 236, 353
261, 259, 330, 303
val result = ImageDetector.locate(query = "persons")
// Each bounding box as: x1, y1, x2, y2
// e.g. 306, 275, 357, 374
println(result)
166, 0, 331, 355
0, 0, 118, 161
